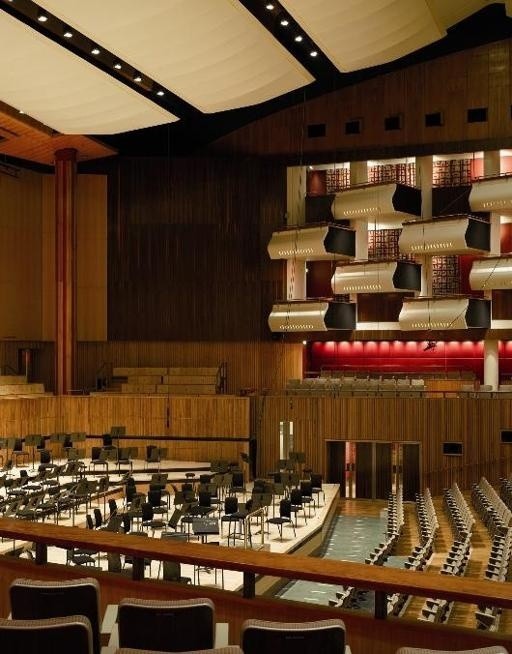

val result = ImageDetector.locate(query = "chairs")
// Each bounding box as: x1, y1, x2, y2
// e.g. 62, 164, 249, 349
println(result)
0, 434, 323, 586
7, 577, 118, 639
107, 595, 230, 652
235, 615, 349, 653
326, 474, 512, 630
1, 614, 95, 653
